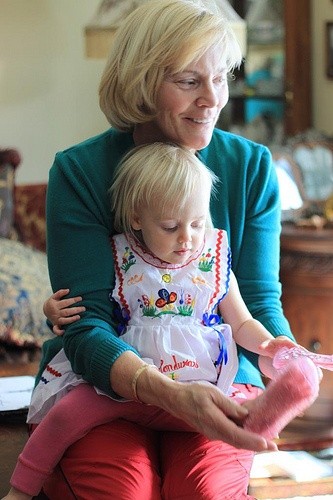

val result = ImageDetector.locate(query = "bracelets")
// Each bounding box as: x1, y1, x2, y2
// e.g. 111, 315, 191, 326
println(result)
132, 362, 159, 406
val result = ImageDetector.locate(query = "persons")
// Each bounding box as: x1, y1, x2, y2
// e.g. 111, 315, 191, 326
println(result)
2, 143, 322, 500
26, 0, 322, 500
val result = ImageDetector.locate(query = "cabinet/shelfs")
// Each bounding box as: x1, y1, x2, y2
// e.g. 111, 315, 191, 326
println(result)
223, 0, 311, 151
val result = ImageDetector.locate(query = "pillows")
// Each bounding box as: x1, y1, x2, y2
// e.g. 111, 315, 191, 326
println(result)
1, 237, 57, 348
